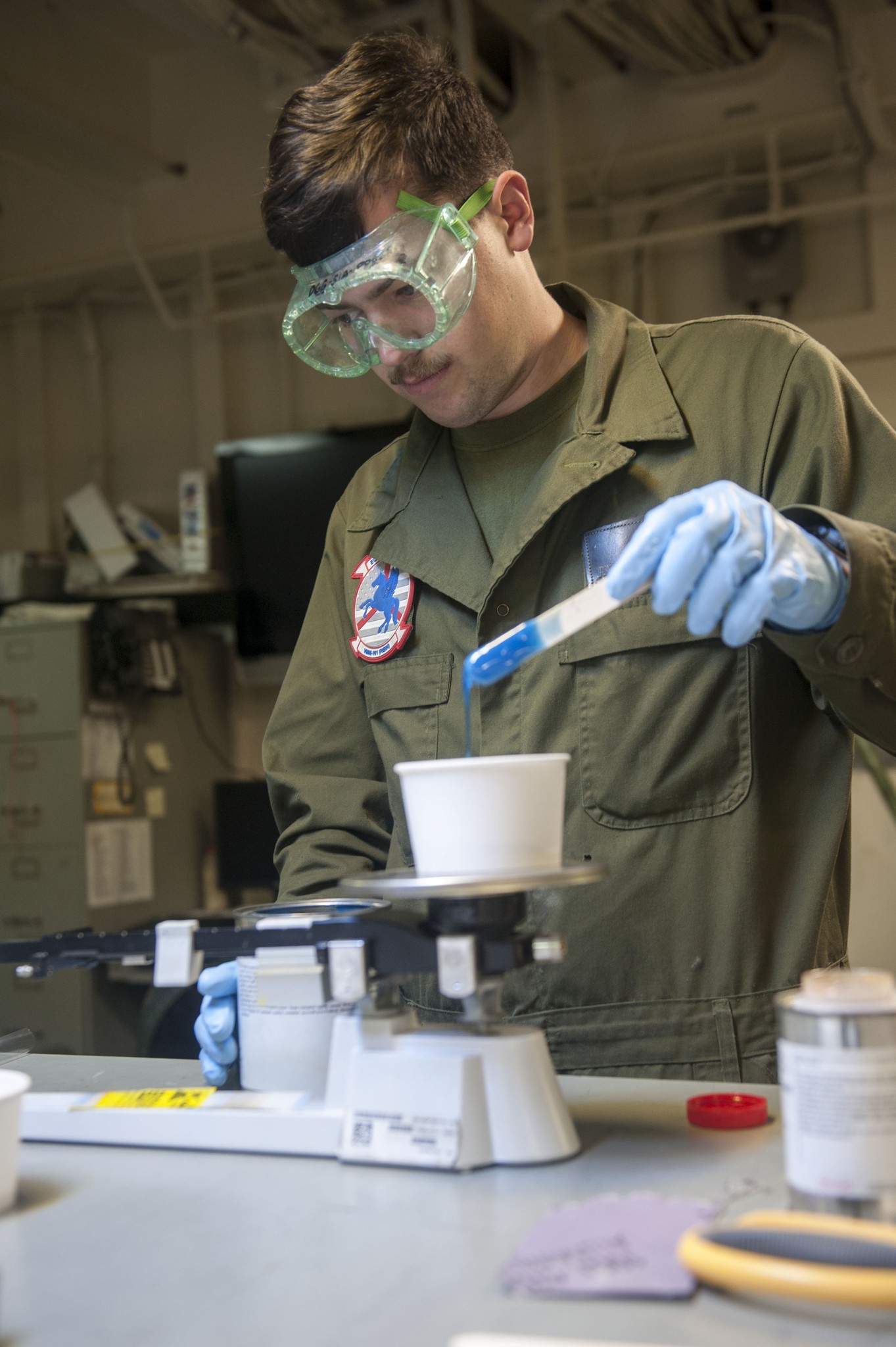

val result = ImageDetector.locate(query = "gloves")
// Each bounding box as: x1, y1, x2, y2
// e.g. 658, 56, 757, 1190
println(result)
193, 960, 238, 1085
609, 480, 842, 652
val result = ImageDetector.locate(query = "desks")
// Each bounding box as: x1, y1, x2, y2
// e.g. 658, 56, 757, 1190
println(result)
1, 1049, 895, 1344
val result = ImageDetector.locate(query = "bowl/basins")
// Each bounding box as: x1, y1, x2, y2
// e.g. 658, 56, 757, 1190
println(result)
393, 753, 571, 877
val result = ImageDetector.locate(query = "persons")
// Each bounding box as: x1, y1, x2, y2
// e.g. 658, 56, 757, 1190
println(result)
263, 36, 896, 1090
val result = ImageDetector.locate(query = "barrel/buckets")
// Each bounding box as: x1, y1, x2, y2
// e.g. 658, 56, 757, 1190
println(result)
231, 895, 392, 1095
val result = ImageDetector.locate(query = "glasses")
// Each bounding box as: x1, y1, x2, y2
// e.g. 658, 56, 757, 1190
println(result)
276, 179, 495, 379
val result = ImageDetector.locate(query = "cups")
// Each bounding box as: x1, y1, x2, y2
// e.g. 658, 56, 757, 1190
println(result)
0, 1067, 33, 1213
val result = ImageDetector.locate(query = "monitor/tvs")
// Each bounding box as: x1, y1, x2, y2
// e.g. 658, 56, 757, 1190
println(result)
209, 779, 283, 891
217, 418, 413, 658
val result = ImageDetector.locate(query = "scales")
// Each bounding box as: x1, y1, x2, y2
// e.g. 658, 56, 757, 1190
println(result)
1, 867, 600, 1171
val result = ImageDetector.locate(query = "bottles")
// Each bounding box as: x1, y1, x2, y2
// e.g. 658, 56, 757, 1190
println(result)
774, 965, 896, 1227
